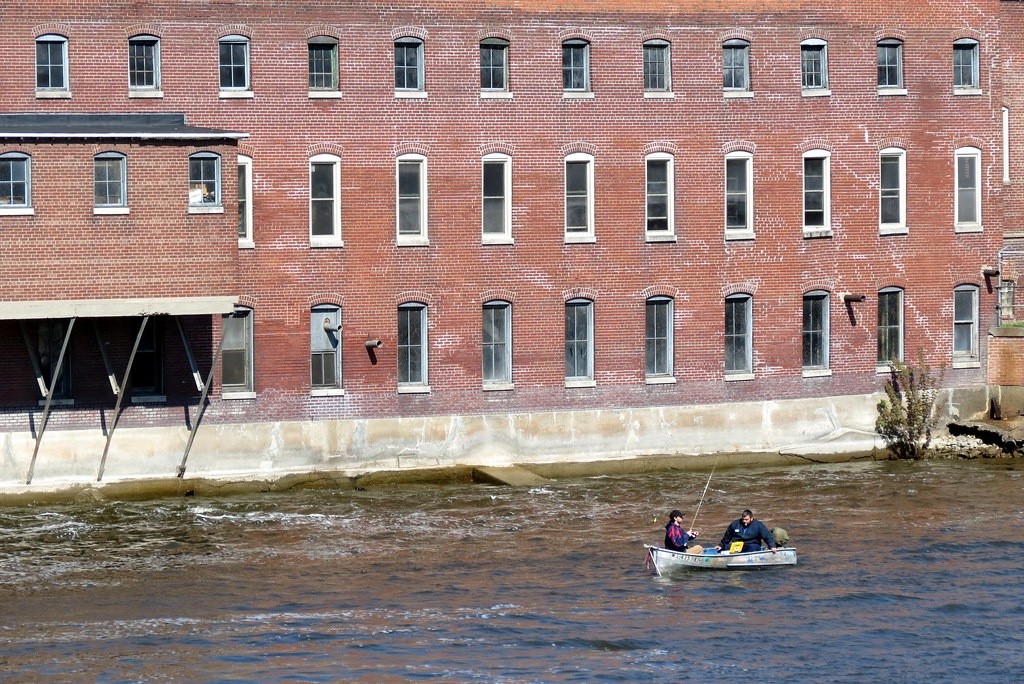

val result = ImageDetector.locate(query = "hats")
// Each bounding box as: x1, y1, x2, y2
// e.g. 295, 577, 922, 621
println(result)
670, 510, 685, 517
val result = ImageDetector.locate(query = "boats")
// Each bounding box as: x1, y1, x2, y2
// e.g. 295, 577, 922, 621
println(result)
644, 543, 797, 574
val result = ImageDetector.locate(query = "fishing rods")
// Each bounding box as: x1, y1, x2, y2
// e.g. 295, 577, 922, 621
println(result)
684, 460, 718, 548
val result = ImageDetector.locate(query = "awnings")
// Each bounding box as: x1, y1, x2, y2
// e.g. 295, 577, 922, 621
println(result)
0, 296, 240, 320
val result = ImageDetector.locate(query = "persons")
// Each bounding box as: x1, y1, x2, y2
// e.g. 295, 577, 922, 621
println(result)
665, 510, 704, 554
714, 510, 777, 555
